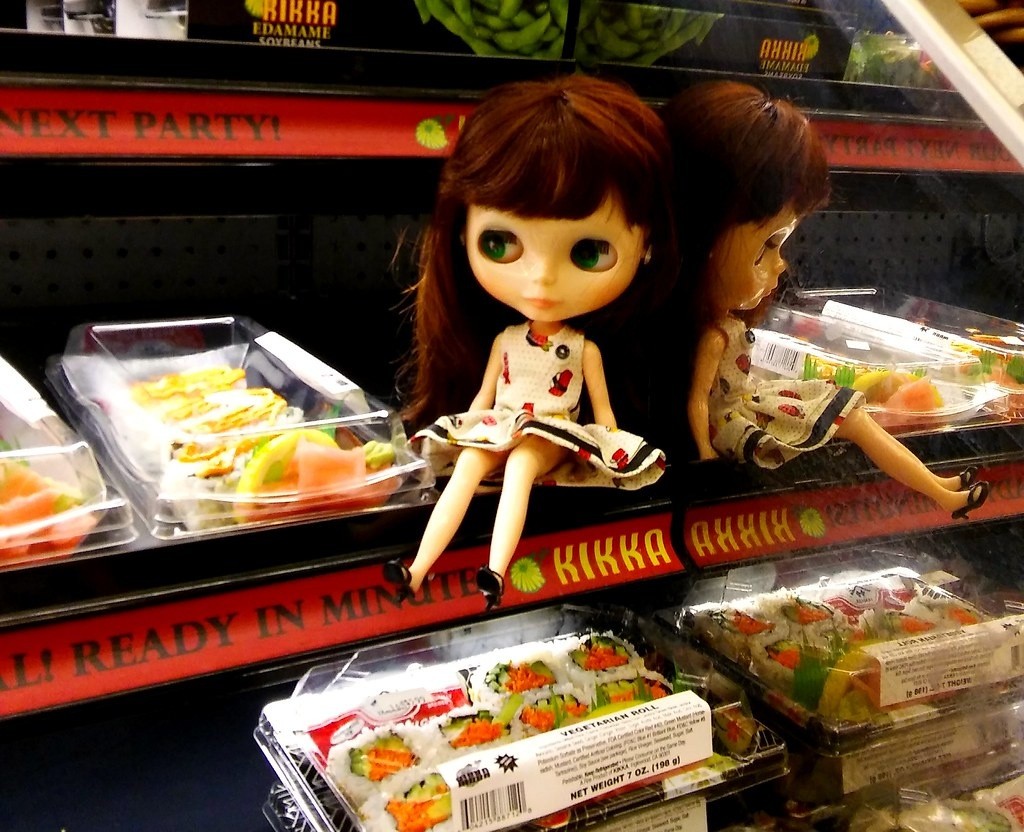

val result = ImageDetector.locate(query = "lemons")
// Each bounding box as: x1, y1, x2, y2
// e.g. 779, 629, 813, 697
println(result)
231, 427, 343, 520
849, 370, 945, 407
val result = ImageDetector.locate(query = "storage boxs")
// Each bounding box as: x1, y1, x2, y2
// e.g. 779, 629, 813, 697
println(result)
677, 585, 1023, 804
186, 0, 573, 59
1, 309, 436, 572
751, 287, 1024, 434
572, 0, 861, 80
254, 594, 790, 832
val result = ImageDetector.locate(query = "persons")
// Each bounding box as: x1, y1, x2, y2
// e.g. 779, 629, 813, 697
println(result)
383, 79, 667, 609
659, 80, 990, 517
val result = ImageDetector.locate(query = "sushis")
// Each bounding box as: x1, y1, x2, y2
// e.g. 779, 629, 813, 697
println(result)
326, 627, 675, 832
695, 577, 980, 707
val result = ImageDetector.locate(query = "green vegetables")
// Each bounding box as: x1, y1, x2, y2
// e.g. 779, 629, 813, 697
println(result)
967, 349, 1024, 385
804, 355, 858, 391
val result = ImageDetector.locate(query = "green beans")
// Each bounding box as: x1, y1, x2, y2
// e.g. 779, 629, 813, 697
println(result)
413, 0, 727, 67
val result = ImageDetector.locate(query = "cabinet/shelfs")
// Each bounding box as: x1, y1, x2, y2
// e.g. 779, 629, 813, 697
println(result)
582, 55, 1024, 580
0, 23, 790, 721
